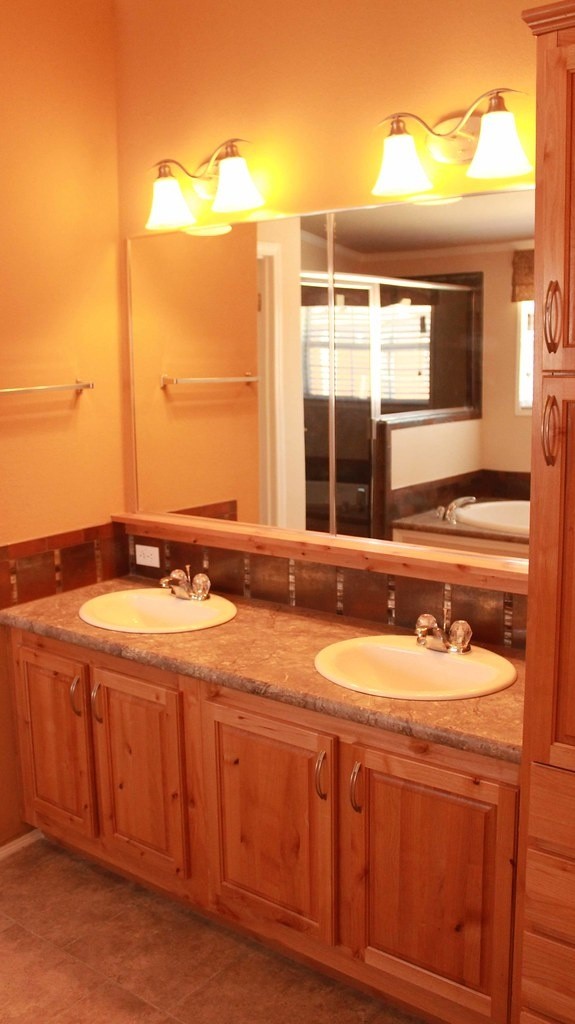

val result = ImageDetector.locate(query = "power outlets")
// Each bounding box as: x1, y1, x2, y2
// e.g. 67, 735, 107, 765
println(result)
135, 544, 160, 569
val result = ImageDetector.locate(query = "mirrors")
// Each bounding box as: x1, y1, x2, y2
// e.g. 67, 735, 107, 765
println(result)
129, 187, 534, 558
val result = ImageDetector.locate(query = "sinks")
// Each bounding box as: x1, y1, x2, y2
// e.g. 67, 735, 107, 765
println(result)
314, 634, 518, 701
455, 500, 530, 537
78, 587, 237, 634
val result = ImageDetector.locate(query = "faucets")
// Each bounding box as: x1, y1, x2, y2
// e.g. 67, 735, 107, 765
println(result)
436, 496, 476, 525
415, 613, 472, 654
159, 569, 211, 601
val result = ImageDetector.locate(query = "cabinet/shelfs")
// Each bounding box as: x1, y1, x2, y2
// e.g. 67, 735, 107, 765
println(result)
9, 626, 192, 910
199, 681, 520, 1024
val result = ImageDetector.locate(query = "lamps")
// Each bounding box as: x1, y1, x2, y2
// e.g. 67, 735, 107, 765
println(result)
143, 137, 259, 232
372, 88, 529, 199
